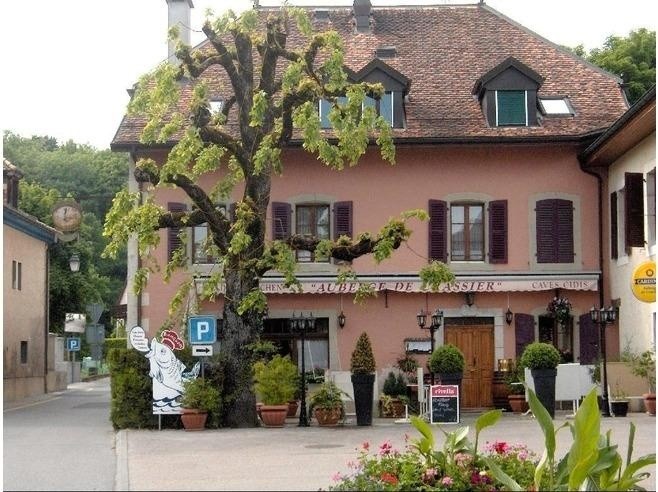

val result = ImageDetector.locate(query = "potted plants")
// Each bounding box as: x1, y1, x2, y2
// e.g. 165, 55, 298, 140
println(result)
248, 330, 420, 426
505, 340, 562, 420
176, 377, 225, 430
609, 383, 630, 418
428, 342, 466, 385
622, 342, 656, 416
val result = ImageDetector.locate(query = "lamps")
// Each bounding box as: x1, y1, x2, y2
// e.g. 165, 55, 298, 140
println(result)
336, 311, 345, 328
505, 308, 514, 326
466, 292, 475, 306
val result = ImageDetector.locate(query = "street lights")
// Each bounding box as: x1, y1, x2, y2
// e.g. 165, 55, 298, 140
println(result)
289, 310, 316, 427
589, 304, 618, 418
417, 309, 443, 385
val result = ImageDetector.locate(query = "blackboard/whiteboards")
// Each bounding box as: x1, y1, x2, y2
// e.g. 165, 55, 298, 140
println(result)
429, 384, 460, 424
